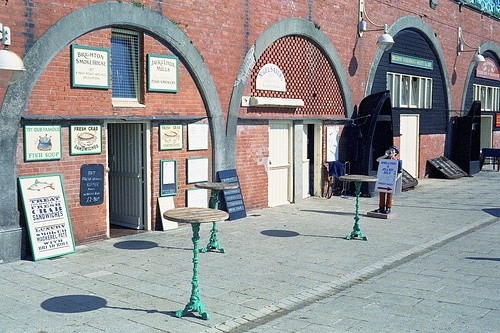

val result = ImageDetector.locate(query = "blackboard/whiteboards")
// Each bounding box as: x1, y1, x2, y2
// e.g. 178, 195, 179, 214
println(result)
80, 163, 105, 206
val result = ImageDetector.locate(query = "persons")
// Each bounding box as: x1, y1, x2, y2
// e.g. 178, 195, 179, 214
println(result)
375, 145, 402, 214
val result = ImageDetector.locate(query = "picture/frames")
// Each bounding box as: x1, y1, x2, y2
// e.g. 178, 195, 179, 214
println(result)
71, 44, 111, 89
147, 53, 178, 95
187, 157, 209, 183
23, 125, 62, 162
187, 124, 208, 150
158, 123, 183, 150
70, 124, 102, 155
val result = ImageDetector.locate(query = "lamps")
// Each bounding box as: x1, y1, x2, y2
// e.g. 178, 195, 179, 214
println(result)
457, 27, 486, 62
358, 0, 395, 45
0, 22, 27, 71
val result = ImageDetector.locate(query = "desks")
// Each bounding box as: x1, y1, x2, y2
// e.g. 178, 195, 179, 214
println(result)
163, 207, 229, 321
195, 182, 238, 253
339, 175, 379, 241
